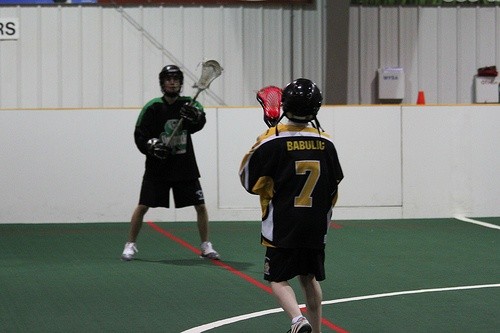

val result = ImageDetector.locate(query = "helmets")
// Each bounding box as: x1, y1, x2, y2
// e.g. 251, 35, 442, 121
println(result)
159, 65, 184, 98
281, 78, 323, 123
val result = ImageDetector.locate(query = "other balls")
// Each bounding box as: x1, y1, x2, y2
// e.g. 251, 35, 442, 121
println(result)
268, 107, 278, 117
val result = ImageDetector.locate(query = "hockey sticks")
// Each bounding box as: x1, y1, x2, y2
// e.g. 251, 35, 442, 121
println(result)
165, 59, 222, 146
256, 85, 283, 128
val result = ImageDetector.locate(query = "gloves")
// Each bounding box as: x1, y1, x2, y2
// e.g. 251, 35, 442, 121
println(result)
179, 103, 198, 122
145, 138, 167, 158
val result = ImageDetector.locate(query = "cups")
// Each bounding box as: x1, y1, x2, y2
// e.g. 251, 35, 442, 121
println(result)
417, 91, 425, 104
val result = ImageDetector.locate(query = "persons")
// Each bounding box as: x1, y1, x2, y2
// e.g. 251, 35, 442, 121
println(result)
239, 77, 345, 333
123, 65, 221, 261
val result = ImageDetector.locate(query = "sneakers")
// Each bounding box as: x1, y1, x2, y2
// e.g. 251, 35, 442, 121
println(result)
121, 242, 139, 259
200, 241, 220, 259
286, 316, 313, 333
